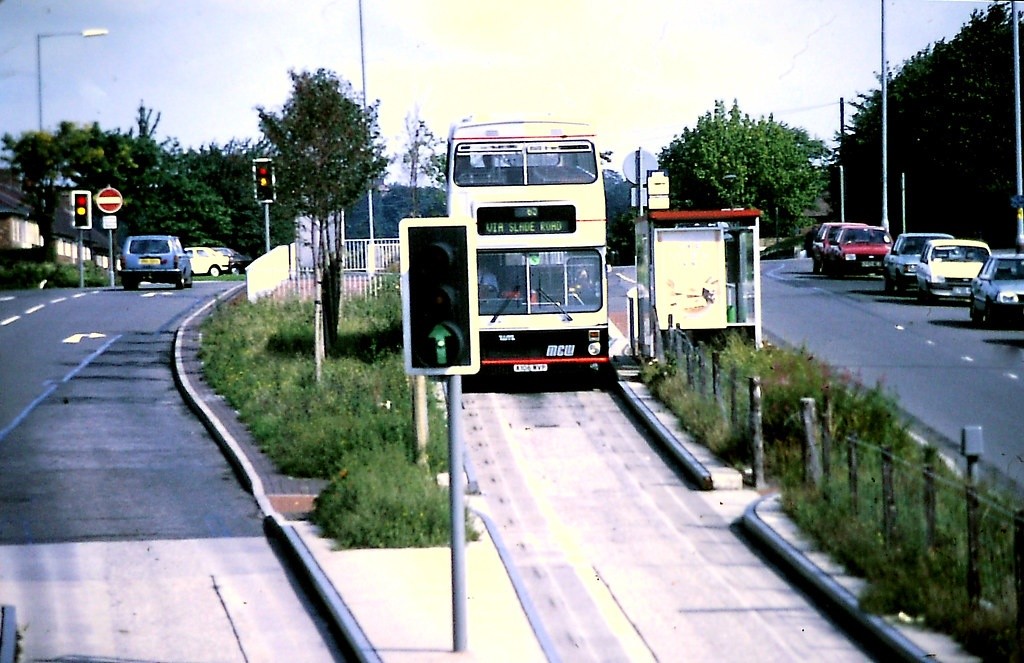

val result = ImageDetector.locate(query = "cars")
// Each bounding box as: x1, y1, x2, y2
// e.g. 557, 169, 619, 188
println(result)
182, 247, 229, 277
811, 222, 865, 273
970, 254, 1024, 325
827, 225, 894, 278
211, 248, 252, 274
883, 232, 955, 292
916, 240, 992, 303
117, 236, 192, 289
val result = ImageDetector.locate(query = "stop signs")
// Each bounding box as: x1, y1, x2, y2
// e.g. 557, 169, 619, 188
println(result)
96, 188, 123, 214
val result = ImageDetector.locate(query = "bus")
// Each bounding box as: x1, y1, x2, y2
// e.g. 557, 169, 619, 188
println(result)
442, 117, 609, 373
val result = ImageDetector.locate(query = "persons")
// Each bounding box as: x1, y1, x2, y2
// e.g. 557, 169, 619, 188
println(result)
477, 261, 500, 294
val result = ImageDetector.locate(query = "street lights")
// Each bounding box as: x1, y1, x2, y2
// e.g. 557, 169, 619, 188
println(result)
37, 29, 108, 132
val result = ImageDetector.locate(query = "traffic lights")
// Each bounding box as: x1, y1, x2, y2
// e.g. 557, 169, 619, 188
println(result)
252, 158, 276, 204
399, 216, 480, 377
70, 190, 92, 230
